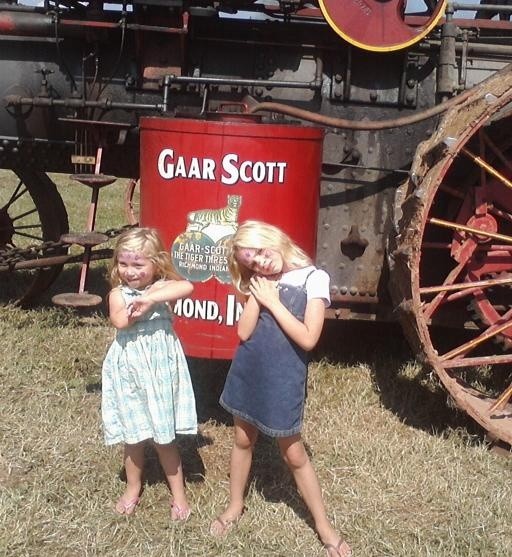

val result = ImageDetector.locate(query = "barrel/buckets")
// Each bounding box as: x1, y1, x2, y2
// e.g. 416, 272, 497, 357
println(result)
139, 115, 324, 360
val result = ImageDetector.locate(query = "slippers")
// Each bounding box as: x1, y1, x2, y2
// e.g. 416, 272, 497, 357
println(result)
116, 497, 139, 517
323, 537, 352, 557
169, 501, 191, 522
210, 517, 236, 538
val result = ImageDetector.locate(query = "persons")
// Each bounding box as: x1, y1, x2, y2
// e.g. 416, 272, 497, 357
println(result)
210, 219, 352, 557
101, 227, 198, 521
